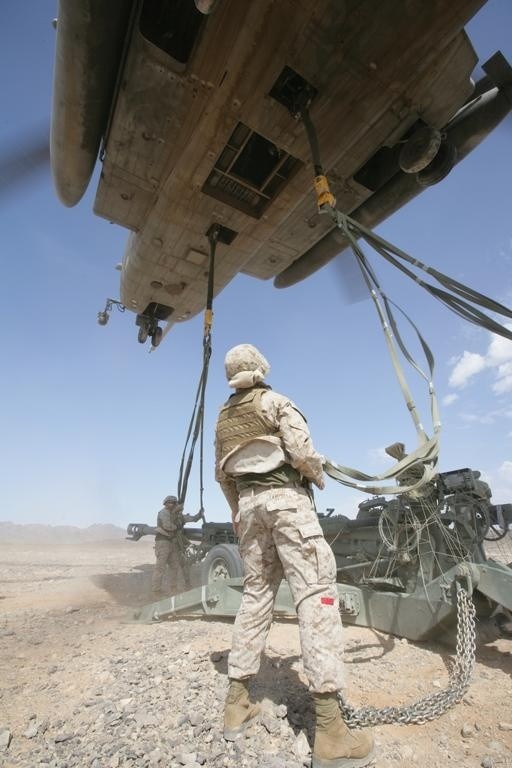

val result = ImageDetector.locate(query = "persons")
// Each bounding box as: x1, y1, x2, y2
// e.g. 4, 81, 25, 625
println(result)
169, 508, 205, 590
151, 495, 185, 601
212, 344, 379, 767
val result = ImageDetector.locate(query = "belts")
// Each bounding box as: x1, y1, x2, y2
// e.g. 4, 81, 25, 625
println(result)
239, 481, 295, 497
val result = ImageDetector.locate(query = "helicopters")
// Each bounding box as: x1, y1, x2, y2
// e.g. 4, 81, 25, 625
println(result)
52, 0, 511, 349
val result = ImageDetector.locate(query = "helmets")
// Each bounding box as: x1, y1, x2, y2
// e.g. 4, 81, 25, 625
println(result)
225, 342, 271, 390
163, 495, 177, 505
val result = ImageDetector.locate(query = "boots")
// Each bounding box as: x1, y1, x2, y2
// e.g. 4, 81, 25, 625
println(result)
312, 694, 372, 761
223, 679, 262, 732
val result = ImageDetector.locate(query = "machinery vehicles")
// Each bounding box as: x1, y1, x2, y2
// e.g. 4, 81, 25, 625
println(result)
127, 468, 512, 591
